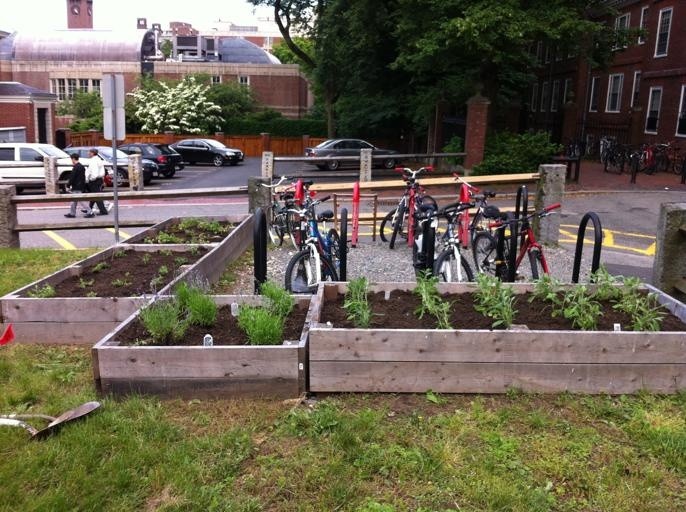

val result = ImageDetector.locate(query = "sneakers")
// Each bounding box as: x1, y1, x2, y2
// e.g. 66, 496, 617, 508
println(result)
63, 208, 109, 218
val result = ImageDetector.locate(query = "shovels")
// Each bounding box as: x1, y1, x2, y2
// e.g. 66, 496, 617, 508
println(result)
0, 401, 101, 442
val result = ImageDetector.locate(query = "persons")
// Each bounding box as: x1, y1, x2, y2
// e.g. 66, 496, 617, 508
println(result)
80, 148, 107, 216
62, 153, 95, 219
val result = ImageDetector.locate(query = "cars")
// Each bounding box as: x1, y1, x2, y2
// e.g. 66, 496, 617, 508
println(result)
0, 143, 183, 194
167, 138, 244, 167
304, 139, 401, 170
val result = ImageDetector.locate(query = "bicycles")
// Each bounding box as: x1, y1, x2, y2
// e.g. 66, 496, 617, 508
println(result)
380, 166, 561, 283
256, 175, 352, 295
566, 137, 686, 175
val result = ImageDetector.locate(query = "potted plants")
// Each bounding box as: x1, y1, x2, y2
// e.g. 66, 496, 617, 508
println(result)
91, 294, 316, 401
308, 281, 684, 400
1, 214, 254, 344
